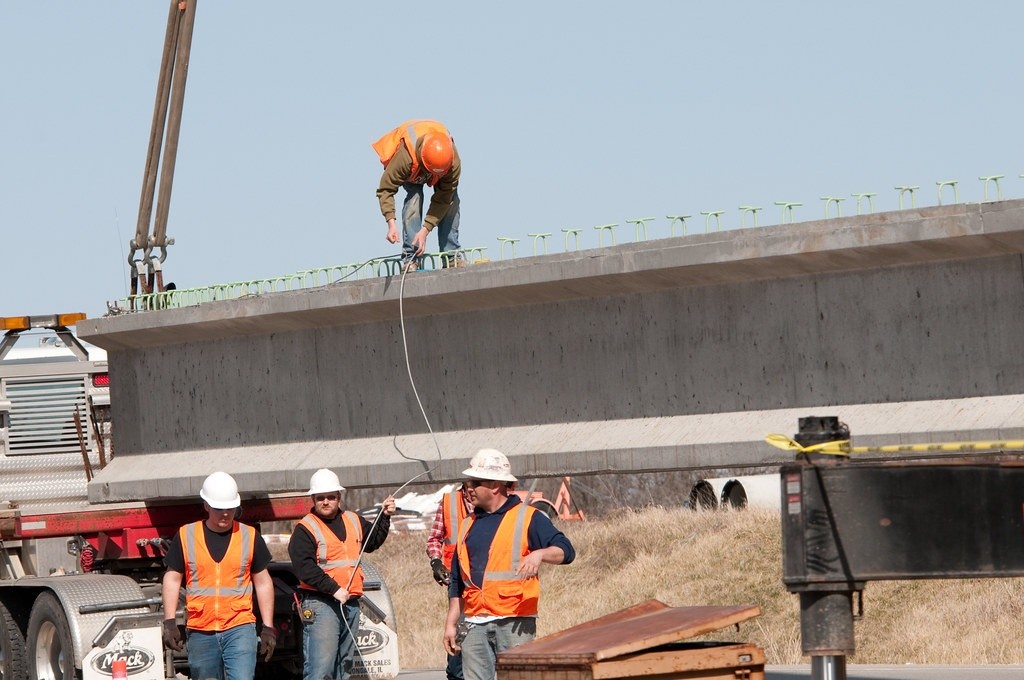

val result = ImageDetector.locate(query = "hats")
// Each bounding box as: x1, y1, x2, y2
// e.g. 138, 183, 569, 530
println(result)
462, 448, 519, 482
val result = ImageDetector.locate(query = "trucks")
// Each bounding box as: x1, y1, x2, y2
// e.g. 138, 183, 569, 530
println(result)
0, 311, 400, 680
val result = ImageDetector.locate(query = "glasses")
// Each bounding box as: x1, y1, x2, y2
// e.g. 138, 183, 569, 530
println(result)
314, 493, 339, 501
470, 478, 496, 487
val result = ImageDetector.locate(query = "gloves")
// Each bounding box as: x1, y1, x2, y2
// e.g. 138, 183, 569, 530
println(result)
260, 623, 276, 661
430, 559, 451, 586
162, 617, 183, 652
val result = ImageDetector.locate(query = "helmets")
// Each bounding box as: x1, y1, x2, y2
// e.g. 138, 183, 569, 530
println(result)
423, 130, 455, 173
307, 468, 346, 494
199, 472, 241, 509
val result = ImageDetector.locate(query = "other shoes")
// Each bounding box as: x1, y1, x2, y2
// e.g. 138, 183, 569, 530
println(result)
402, 262, 420, 273
445, 260, 465, 269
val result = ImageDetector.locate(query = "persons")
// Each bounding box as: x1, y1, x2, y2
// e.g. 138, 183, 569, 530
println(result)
443, 449, 575, 680
372, 120, 466, 273
426, 480, 477, 680
162, 471, 277, 680
288, 468, 396, 680
